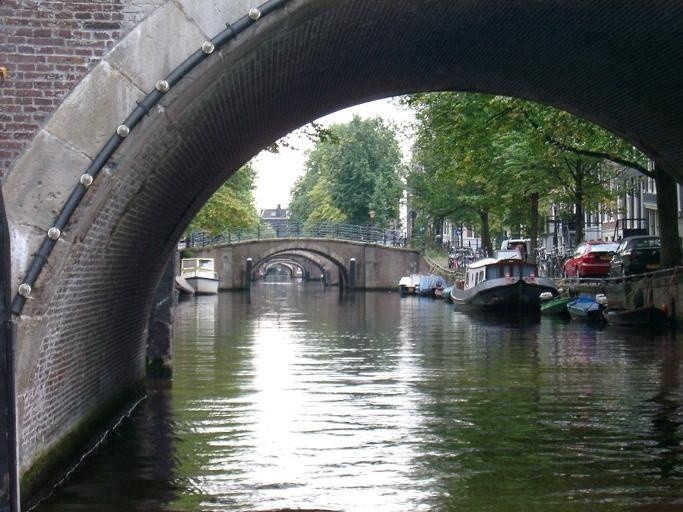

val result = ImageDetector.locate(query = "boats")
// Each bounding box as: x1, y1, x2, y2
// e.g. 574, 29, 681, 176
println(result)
450, 250, 560, 312
398, 274, 451, 302
540, 295, 669, 328
181, 258, 219, 296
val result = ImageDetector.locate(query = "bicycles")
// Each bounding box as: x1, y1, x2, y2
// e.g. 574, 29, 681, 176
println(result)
448, 235, 682, 282
